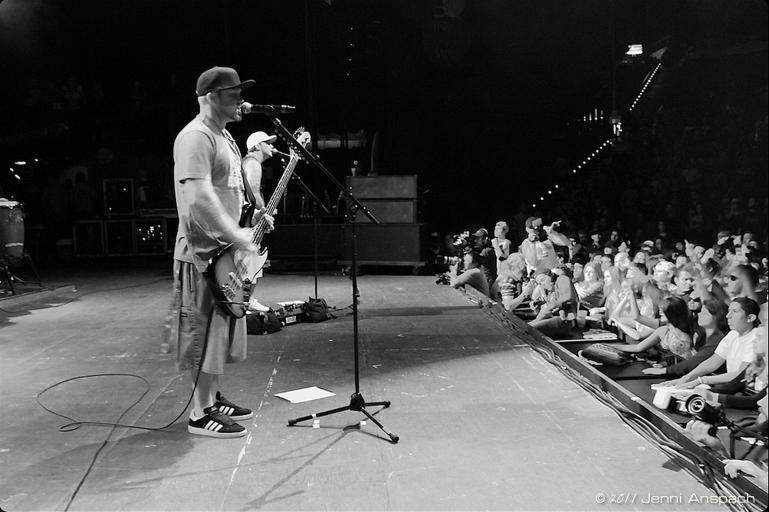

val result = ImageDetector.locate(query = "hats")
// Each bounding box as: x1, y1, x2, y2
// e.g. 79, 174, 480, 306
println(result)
496, 221, 510, 232
640, 239, 654, 248
246, 130, 278, 151
196, 65, 256, 98
471, 227, 489, 238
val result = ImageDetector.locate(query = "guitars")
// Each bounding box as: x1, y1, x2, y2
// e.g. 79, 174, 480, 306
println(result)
207, 126, 313, 319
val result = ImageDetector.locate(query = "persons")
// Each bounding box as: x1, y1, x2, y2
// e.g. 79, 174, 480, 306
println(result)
168, 65, 278, 441
446, 197, 768, 497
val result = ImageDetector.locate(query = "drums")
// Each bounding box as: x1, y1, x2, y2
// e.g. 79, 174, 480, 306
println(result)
0, 205, 25, 262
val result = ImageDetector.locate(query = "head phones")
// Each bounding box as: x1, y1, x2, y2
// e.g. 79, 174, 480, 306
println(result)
480, 228, 491, 245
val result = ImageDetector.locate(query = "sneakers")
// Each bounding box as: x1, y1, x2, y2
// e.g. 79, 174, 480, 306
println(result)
245, 297, 273, 313
187, 391, 253, 438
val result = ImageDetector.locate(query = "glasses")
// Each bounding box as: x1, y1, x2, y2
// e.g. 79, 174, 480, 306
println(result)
723, 273, 740, 281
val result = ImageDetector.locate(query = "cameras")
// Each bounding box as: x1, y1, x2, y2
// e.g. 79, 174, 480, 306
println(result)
436, 275, 447, 285
685, 394, 725, 437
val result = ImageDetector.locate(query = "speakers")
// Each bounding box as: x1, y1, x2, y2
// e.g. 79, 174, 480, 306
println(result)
344, 175, 418, 224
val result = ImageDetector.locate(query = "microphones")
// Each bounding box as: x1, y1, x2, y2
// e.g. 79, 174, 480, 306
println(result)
272, 148, 302, 160
241, 102, 296, 114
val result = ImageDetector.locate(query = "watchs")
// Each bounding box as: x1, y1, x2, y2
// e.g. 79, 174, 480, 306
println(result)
234, 131, 280, 316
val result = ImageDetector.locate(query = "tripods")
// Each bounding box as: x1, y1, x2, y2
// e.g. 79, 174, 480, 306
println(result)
288, 206, 400, 443
717, 424, 769, 458
284, 199, 339, 320
